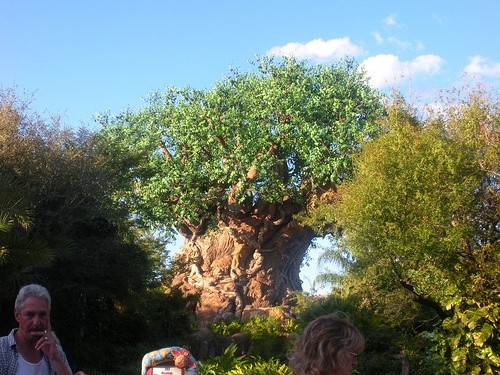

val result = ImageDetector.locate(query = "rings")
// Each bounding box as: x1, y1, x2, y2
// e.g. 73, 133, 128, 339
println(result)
42, 330, 47, 334
43, 337, 48, 341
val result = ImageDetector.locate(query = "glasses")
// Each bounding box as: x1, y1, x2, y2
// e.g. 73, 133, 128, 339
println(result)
349, 352, 358, 359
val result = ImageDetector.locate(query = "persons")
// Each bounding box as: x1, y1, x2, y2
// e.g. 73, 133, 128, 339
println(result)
0, 284, 72, 375
289, 311, 365, 375
